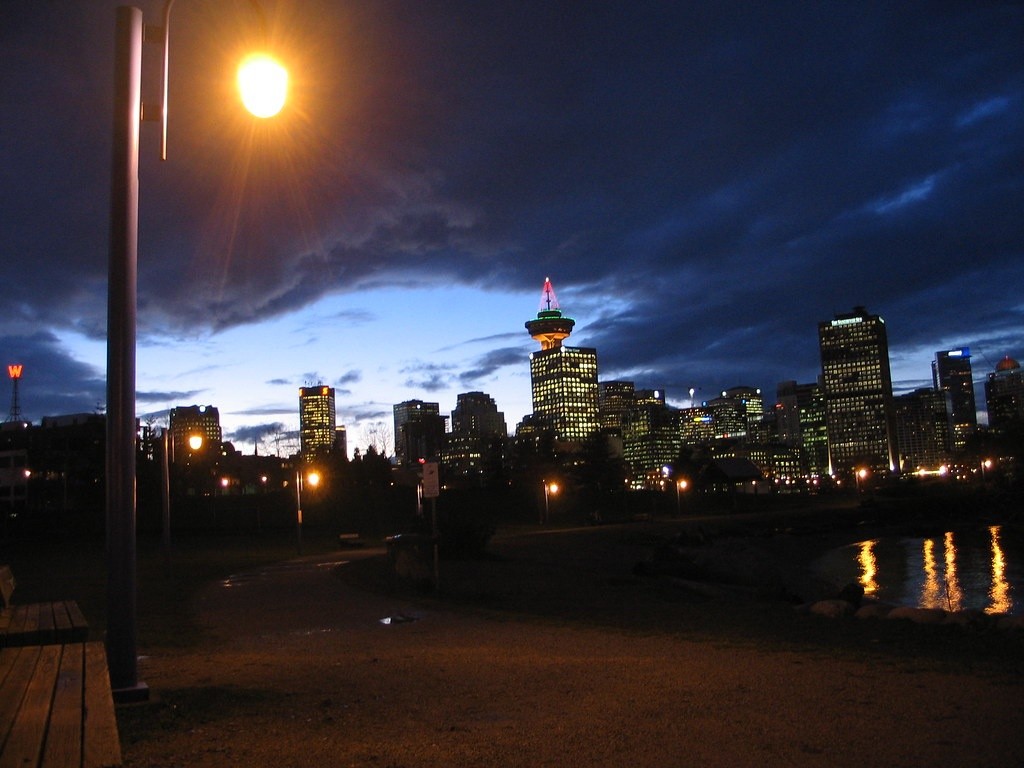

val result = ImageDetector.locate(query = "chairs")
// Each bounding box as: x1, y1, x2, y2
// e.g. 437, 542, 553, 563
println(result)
0, 564, 91, 645
0, 640, 122, 768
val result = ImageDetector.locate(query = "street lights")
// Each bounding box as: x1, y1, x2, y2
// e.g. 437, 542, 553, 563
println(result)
854, 467, 868, 495
542, 478, 559, 527
100, 0, 291, 706
157, 424, 203, 563
290, 466, 322, 558
676, 479, 690, 517
